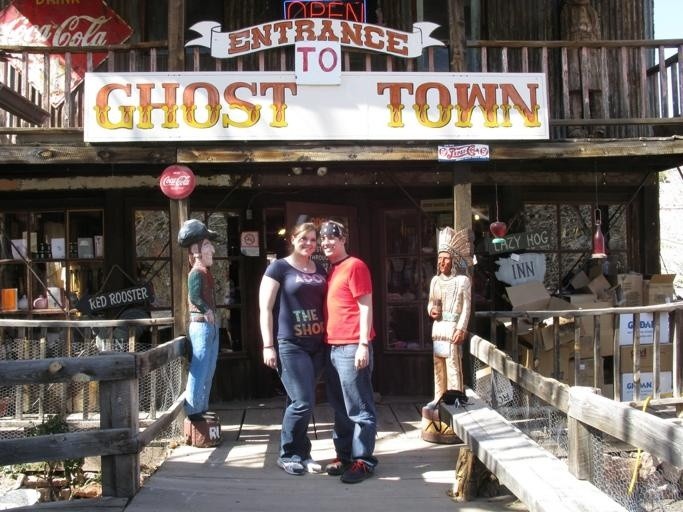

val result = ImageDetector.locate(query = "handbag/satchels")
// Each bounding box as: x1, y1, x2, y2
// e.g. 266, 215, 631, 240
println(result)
431, 390, 468, 432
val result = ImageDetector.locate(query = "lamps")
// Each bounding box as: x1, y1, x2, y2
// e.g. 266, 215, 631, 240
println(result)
588, 158, 610, 260
488, 161, 510, 247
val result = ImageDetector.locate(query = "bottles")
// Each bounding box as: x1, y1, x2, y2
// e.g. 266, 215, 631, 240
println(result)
593, 218, 604, 254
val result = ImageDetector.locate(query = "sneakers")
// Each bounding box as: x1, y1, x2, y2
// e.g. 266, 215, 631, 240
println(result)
277, 455, 305, 475
302, 457, 322, 474
340, 461, 374, 483
326, 457, 348, 476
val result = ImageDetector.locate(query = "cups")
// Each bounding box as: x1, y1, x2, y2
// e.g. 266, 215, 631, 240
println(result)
50, 237, 66, 259
0, 288, 18, 313
45, 287, 62, 309
10, 239, 27, 260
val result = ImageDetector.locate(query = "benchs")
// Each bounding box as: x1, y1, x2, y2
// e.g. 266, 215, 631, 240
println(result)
435, 329, 683, 512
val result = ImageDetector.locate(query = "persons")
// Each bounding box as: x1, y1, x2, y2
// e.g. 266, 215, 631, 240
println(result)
175, 219, 228, 422
316, 214, 380, 484
259, 219, 331, 476
422, 226, 479, 410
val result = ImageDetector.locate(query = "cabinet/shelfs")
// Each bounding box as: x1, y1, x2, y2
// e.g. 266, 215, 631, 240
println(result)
0, 207, 135, 318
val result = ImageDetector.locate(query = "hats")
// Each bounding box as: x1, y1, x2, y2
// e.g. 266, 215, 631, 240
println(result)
178, 218, 219, 247
319, 219, 345, 239
436, 225, 474, 270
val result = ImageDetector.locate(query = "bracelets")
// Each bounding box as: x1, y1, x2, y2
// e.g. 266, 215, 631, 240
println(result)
262, 344, 275, 348
359, 342, 369, 348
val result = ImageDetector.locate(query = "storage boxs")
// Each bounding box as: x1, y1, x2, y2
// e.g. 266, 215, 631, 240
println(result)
493, 268, 682, 416
